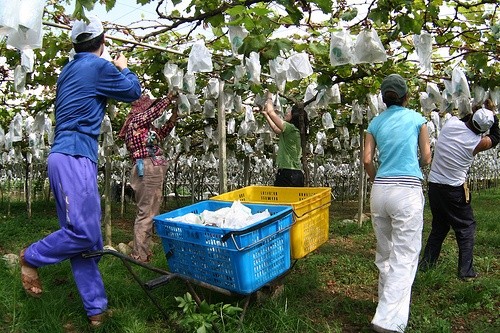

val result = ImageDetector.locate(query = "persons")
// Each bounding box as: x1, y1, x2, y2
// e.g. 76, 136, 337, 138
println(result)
262, 89, 307, 187
365, 74, 431, 333
21, 16, 141, 325
119, 91, 178, 262
421, 101, 500, 281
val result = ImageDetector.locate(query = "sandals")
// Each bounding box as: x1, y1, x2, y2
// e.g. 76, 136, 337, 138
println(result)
19, 247, 43, 298
88, 309, 114, 328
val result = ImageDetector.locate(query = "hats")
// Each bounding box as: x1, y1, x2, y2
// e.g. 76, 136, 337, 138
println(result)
129, 95, 157, 115
72, 15, 105, 44
380, 73, 408, 99
471, 107, 495, 133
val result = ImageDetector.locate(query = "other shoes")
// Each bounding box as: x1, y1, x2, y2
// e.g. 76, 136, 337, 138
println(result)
370, 323, 401, 333
458, 272, 480, 282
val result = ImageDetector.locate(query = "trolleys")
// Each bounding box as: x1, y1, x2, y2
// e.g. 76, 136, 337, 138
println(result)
81, 238, 322, 333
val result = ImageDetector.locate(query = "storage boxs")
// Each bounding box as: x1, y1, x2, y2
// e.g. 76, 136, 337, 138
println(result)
209, 186, 336, 259
152, 200, 297, 294
